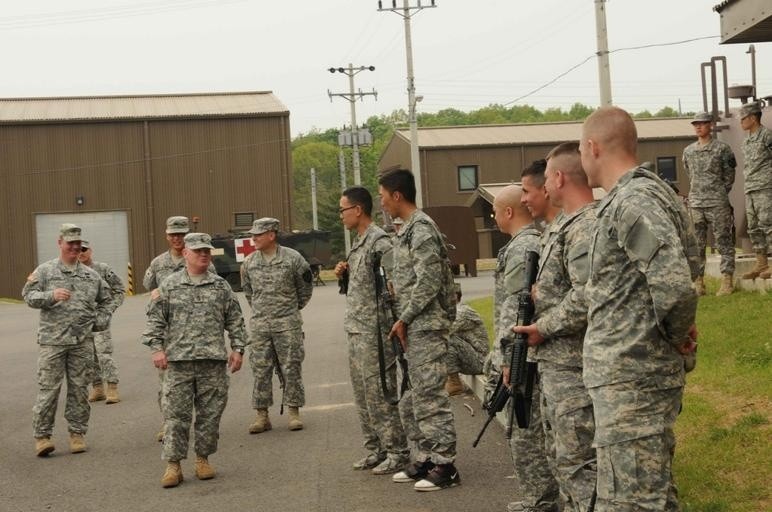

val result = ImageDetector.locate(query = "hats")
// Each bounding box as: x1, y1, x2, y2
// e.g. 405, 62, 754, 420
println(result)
734, 101, 761, 120
690, 113, 717, 124
58, 215, 280, 251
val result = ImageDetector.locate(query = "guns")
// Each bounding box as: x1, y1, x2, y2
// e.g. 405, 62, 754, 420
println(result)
472, 250, 535, 449
378, 266, 405, 370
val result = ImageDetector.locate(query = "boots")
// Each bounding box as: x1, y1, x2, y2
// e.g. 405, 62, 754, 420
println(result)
716, 272, 733, 296
88, 384, 106, 402
36, 437, 55, 456
194, 452, 216, 479
445, 372, 465, 397
69, 432, 87, 453
743, 255, 772, 279
105, 383, 120, 403
694, 276, 705, 297
160, 461, 184, 486
288, 407, 303, 431
249, 408, 272, 433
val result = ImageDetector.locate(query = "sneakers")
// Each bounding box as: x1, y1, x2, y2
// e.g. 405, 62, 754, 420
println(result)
351, 451, 462, 492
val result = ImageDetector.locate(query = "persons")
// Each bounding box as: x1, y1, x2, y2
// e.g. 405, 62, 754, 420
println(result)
77, 238, 124, 404
389, 216, 404, 239
444, 281, 491, 396
140, 232, 248, 486
376, 168, 463, 492
142, 215, 217, 442
21, 222, 111, 457
483, 104, 699, 511
334, 185, 410, 475
239, 217, 313, 434
682, 111, 738, 297
739, 101, 772, 279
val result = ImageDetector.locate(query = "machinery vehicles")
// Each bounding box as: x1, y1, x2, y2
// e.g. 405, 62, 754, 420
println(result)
204, 227, 334, 292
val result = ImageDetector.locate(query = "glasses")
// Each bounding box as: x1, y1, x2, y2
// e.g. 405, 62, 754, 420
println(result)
339, 205, 357, 213
